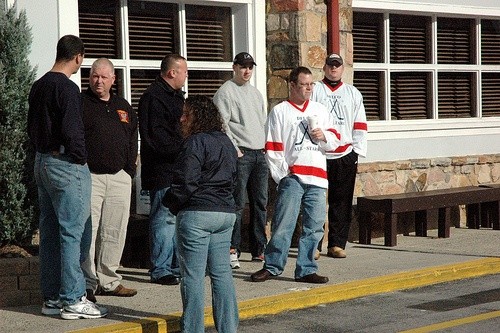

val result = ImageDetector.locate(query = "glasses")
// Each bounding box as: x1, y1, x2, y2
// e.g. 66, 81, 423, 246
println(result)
297, 82, 316, 87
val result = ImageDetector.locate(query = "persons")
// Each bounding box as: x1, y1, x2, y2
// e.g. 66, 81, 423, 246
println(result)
138, 54, 190, 286
310, 54, 367, 260
165, 94, 241, 333
28, 35, 110, 320
210, 52, 270, 269
74, 56, 138, 303
250, 66, 341, 283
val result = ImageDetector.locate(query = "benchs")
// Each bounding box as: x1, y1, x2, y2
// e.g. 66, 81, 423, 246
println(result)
478, 184, 499, 230
356, 184, 499, 249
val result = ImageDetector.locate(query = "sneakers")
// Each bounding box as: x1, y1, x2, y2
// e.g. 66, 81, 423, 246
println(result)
40, 297, 64, 315
61, 296, 109, 320
230, 253, 240, 269
252, 255, 266, 262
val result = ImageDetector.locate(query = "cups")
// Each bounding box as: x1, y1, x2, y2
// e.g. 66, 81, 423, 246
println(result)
306, 114, 320, 135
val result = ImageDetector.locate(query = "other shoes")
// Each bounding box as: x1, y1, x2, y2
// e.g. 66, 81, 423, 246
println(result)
86, 289, 96, 303
314, 249, 320, 260
327, 246, 346, 258
100, 284, 137, 297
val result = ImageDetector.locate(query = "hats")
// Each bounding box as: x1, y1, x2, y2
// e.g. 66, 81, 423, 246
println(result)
234, 52, 257, 66
326, 53, 343, 64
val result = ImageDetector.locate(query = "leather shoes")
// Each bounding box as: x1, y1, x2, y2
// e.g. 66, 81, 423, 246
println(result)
251, 269, 277, 282
295, 273, 329, 283
154, 275, 179, 285
